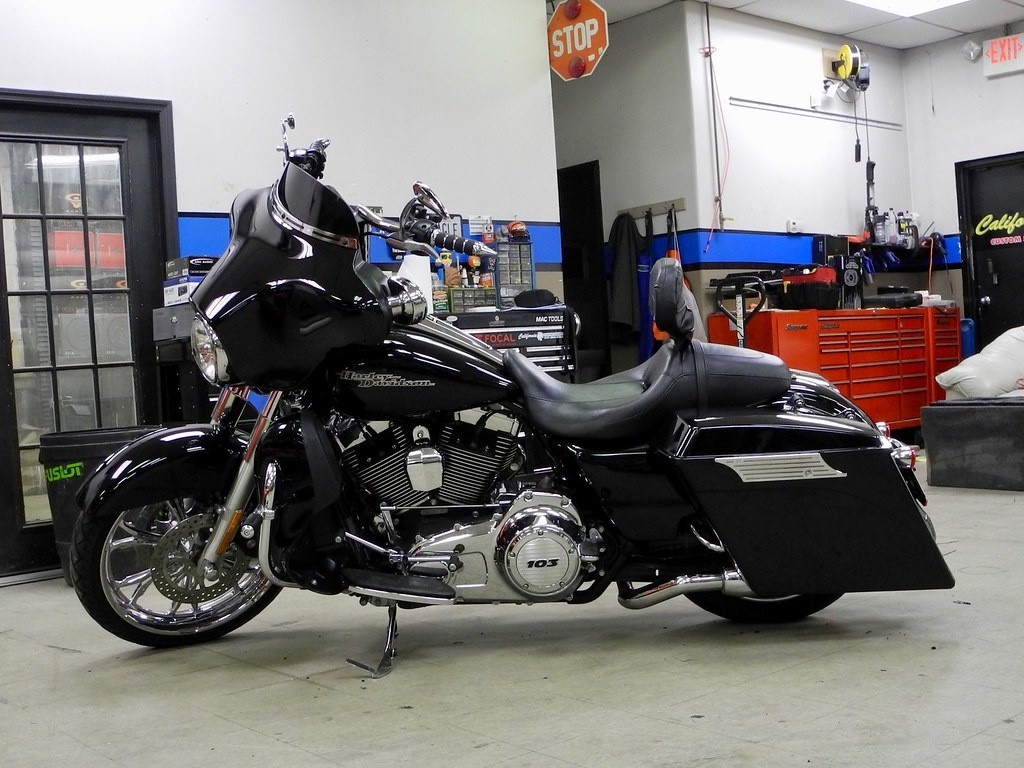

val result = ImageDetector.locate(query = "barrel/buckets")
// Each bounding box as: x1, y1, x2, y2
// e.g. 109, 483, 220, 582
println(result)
40, 425, 165, 587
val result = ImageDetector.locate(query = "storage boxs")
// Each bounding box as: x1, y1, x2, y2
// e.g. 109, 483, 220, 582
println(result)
165, 255, 219, 278
153, 303, 199, 341
163, 275, 206, 308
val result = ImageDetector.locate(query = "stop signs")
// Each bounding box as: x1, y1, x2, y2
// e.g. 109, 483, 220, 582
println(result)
547, 0, 609, 82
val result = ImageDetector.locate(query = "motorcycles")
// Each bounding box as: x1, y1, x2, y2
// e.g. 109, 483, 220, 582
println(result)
69, 112, 956, 679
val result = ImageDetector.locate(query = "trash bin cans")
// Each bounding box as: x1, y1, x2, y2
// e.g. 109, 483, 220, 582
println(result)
36, 426, 167, 587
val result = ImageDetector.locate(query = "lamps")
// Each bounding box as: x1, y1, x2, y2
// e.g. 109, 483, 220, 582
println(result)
960, 39, 981, 60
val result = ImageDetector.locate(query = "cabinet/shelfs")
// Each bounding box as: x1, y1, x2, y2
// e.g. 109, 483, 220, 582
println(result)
706, 303, 963, 432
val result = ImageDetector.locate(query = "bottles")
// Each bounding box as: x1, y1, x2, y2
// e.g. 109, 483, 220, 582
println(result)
884, 208, 913, 244
